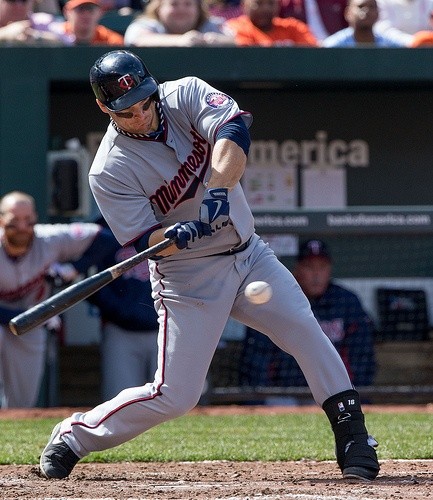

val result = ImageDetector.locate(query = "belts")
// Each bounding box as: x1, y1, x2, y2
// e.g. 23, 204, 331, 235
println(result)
221, 239, 251, 255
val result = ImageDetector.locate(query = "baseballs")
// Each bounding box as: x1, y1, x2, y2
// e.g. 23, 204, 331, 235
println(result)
244, 279, 272, 305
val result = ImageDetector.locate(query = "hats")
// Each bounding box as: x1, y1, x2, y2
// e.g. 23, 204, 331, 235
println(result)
300, 238, 329, 260
64, 0, 104, 10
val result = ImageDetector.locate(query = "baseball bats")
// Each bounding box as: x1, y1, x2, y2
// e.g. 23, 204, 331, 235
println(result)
7, 236, 179, 337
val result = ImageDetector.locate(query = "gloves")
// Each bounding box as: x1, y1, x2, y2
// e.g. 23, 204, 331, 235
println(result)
164, 220, 203, 253
199, 188, 230, 237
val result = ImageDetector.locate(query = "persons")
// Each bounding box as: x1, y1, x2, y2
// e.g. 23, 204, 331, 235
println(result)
0, 192, 387, 408
0, 0, 433, 48
38, 51, 379, 480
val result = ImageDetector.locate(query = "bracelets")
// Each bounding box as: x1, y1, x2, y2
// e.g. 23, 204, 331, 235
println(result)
34, 30, 43, 46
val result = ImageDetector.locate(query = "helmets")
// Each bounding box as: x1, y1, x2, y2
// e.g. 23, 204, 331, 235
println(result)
89, 49, 157, 111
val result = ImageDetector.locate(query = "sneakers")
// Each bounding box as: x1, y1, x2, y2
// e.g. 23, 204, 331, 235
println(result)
40, 422, 80, 480
342, 467, 378, 481
